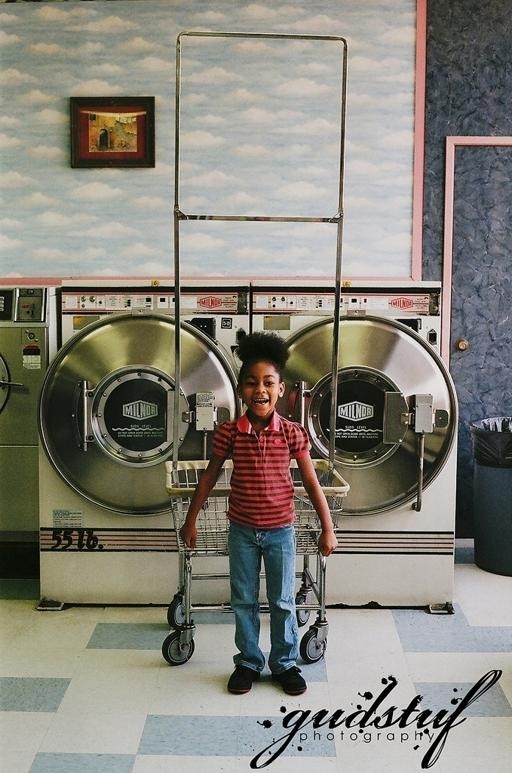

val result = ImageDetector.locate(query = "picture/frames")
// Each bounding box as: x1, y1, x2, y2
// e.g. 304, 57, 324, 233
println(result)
68, 96, 155, 170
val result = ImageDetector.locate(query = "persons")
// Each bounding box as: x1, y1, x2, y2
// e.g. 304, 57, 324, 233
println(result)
179, 332, 339, 696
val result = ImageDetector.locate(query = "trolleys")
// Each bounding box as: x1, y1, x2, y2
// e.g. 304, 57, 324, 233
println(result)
162, 452, 352, 666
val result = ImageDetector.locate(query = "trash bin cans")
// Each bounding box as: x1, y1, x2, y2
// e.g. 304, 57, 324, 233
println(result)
469, 416, 512, 577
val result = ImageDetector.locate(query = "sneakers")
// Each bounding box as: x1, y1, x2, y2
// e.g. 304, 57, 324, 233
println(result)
227, 664, 260, 694
272, 665, 307, 695
